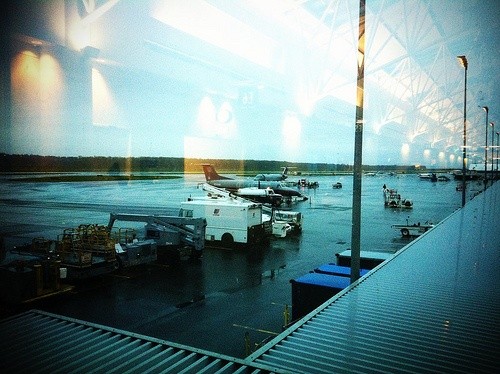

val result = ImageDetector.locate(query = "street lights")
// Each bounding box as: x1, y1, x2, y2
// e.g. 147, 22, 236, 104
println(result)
490, 122, 500, 185
458, 55, 467, 205
482, 106, 488, 188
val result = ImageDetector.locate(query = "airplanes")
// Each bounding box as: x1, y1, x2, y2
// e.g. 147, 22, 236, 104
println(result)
200, 164, 302, 197
254, 167, 289, 181
